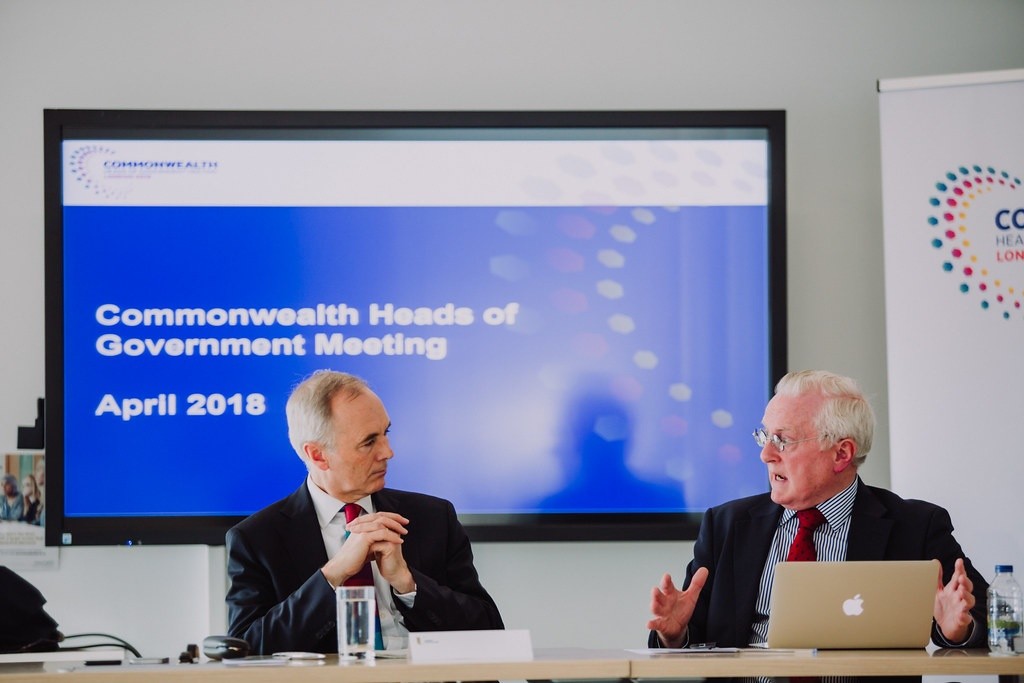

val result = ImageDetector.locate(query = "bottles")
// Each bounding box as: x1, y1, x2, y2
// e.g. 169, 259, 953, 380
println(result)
986, 565, 1023, 656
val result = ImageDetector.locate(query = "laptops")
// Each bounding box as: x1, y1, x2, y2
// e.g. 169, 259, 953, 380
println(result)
748, 561, 940, 648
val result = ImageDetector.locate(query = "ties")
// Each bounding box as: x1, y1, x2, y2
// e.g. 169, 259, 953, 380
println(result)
785, 507, 829, 683
342, 505, 378, 617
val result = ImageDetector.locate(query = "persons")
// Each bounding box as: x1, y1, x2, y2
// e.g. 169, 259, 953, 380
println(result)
224, 368, 506, 656
0, 455, 46, 528
645, 368, 991, 649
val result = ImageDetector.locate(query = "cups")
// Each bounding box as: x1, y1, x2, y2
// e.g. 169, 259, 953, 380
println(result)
335, 586, 376, 663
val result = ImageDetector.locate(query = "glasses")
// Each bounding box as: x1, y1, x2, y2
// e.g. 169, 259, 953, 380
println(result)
751, 428, 828, 452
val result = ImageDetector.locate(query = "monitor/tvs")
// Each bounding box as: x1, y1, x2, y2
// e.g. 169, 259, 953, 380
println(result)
42, 107, 791, 550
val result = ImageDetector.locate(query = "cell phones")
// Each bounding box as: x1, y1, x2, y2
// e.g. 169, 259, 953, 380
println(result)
84, 660, 121, 665
129, 657, 169, 664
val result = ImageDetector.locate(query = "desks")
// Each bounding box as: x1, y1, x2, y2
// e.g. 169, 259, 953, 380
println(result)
586, 648, 1022, 682
2, 644, 630, 683
